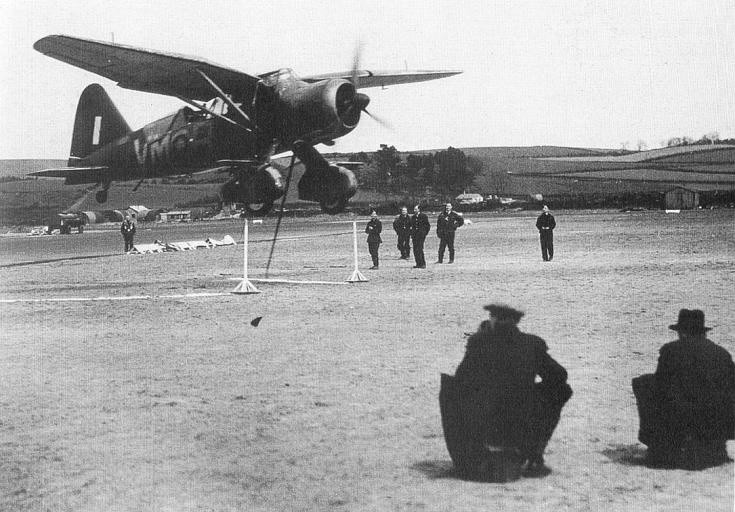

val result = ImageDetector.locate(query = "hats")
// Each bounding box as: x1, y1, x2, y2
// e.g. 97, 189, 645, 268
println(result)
667, 309, 712, 333
482, 305, 525, 322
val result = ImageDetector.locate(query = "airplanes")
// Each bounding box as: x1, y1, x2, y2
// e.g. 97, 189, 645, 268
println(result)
26, 27, 463, 218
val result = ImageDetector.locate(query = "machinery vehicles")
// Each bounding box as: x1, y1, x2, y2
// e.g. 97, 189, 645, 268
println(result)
46, 212, 85, 233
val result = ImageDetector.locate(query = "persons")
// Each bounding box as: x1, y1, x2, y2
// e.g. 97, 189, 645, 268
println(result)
364, 211, 382, 269
632, 306, 735, 469
439, 303, 574, 483
393, 206, 410, 259
408, 204, 431, 269
120, 216, 138, 253
536, 205, 557, 260
435, 203, 465, 265
